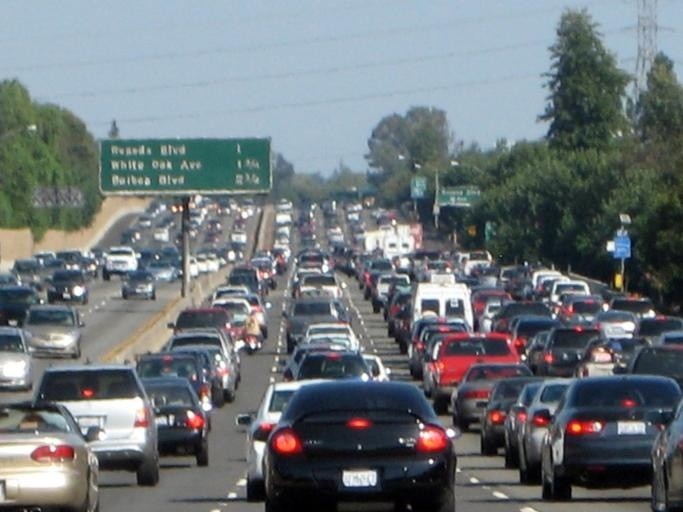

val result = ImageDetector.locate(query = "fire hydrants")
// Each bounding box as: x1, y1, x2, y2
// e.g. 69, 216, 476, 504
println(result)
398, 154, 419, 212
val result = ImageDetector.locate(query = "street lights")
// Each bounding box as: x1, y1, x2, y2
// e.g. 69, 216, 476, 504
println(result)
0, 124, 36, 141
450, 160, 497, 182
412, 162, 438, 228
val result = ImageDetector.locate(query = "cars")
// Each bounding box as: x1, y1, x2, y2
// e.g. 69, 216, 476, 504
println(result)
249, 379, 461, 512
0, 402, 106, 512
642, 391, 683, 512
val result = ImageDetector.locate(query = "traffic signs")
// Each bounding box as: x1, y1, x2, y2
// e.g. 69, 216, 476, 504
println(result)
439, 188, 480, 206
98, 136, 271, 195
